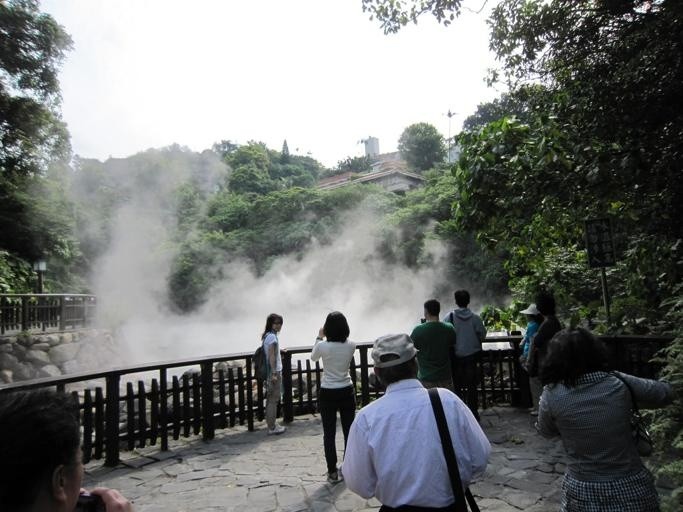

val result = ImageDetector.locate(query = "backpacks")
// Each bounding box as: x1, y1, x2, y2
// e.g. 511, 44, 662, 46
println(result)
253, 333, 278, 383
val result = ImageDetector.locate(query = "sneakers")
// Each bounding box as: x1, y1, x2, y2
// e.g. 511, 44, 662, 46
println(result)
528, 407, 538, 416
267, 423, 286, 435
327, 473, 339, 483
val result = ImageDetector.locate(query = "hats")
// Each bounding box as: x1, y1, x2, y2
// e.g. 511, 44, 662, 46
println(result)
519, 304, 540, 315
371, 333, 419, 369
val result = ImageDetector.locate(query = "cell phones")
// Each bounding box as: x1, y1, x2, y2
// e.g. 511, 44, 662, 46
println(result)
421, 319, 426, 324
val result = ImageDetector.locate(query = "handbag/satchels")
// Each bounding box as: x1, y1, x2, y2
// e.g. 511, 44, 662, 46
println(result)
631, 422, 652, 457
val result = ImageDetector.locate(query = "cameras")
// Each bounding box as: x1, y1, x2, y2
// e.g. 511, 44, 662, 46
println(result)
74, 493, 106, 512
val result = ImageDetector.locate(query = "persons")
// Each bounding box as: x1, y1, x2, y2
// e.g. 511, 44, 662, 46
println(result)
310, 311, 357, 479
262, 313, 286, 435
339, 333, 492, 512
444, 290, 486, 421
0, 390, 134, 512
410, 300, 456, 390
518, 302, 541, 414
535, 293, 561, 386
534, 328, 675, 512
